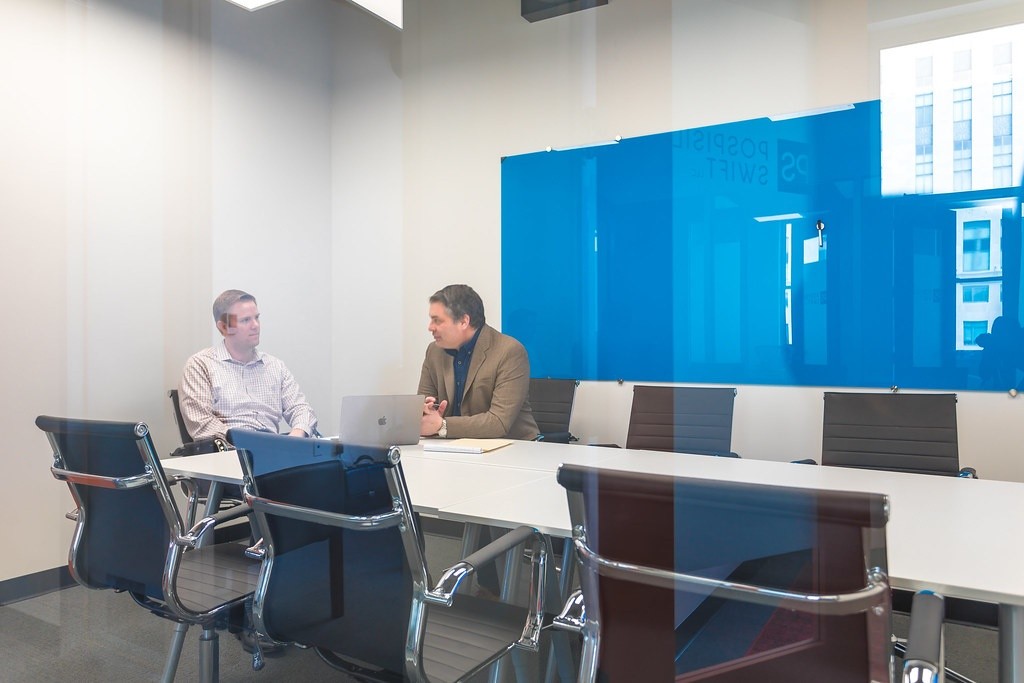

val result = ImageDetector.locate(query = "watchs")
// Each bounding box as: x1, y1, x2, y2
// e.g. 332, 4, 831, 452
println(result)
438, 418, 447, 439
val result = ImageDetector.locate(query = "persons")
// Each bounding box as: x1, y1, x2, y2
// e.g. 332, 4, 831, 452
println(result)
419, 284, 540, 440
178, 289, 318, 439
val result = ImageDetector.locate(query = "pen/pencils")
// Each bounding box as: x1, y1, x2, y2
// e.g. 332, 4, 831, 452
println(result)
425, 403, 439, 408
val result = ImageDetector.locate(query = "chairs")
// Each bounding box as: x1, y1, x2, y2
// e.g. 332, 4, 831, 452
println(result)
35, 415, 266, 683
168, 390, 254, 546
553, 462, 945, 683
589, 385, 738, 458
526, 379, 580, 445
225, 428, 547, 683
790, 392, 976, 479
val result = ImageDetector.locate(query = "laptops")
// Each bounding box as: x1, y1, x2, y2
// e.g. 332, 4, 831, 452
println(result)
339, 394, 425, 446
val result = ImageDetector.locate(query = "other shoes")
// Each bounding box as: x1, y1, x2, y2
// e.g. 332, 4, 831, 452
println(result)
471, 584, 499, 601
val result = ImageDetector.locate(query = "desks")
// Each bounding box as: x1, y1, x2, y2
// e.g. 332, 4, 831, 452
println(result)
156, 418, 1024, 683
